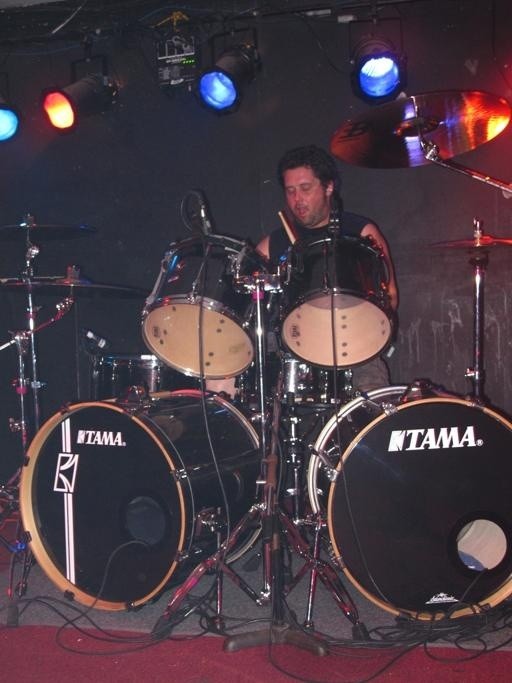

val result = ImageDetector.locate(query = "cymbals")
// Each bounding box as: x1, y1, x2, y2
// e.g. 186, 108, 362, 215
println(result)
330, 89, 512, 167
1, 221, 93, 239
1, 274, 138, 296
427, 235, 512, 252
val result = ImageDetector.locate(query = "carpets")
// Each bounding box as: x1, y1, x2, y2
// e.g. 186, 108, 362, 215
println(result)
0, 623, 512, 683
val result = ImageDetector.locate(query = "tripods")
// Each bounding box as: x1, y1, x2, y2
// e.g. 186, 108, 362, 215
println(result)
147, 272, 371, 659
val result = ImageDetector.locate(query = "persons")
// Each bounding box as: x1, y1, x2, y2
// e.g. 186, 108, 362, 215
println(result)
256, 146, 396, 416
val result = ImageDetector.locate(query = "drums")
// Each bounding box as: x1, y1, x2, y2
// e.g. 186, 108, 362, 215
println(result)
91, 354, 200, 399
139, 232, 276, 383
18, 389, 263, 613
307, 383, 510, 620
247, 358, 354, 410
279, 235, 397, 370
